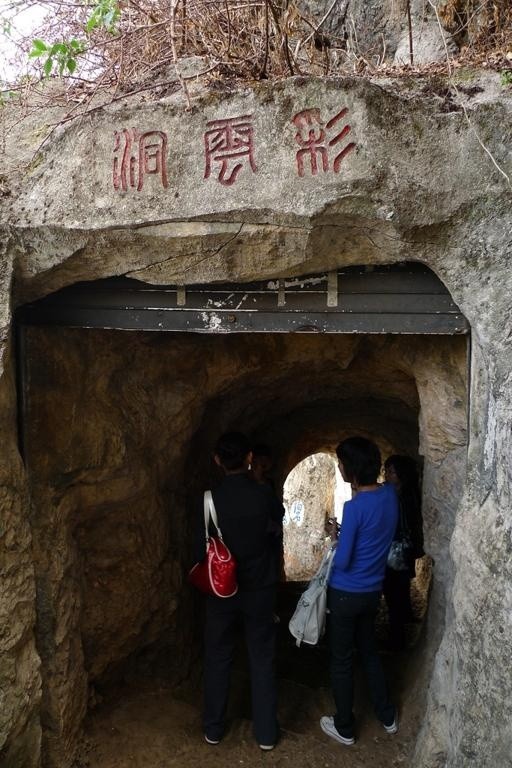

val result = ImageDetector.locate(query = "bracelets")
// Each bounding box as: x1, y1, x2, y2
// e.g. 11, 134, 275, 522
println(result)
329, 529, 338, 534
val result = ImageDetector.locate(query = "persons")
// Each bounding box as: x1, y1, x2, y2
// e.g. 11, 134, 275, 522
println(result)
319, 436, 402, 747
189, 431, 286, 753
378, 453, 426, 663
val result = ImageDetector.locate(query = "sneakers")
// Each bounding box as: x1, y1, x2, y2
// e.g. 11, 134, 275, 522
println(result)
259, 741, 277, 751
204, 735, 219, 745
383, 708, 399, 733
320, 716, 356, 746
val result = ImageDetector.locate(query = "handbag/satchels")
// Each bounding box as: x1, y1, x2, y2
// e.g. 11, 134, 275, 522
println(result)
288, 559, 331, 645
185, 536, 239, 599
